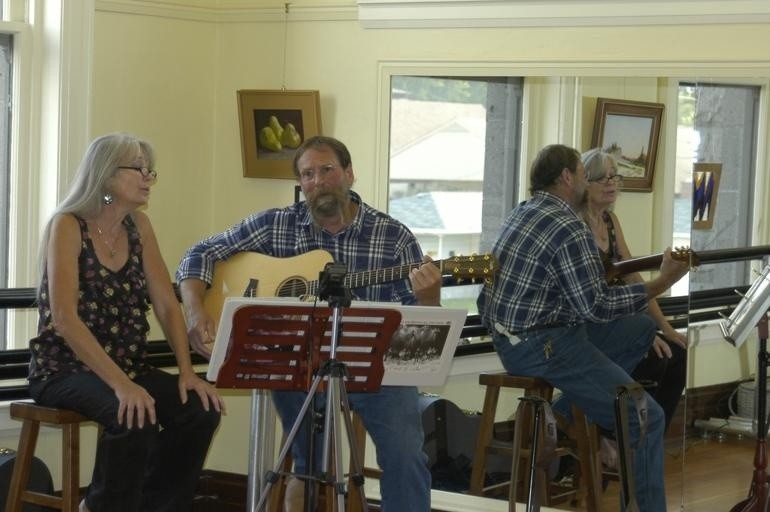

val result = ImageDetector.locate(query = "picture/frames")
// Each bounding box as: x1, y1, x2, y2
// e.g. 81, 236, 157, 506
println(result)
235, 88, 323, 180
693, 162, 724, 230
295, 186, 306, 203
593, 98, 663, 193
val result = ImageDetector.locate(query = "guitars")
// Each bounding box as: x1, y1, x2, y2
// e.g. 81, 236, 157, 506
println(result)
594, 245, 703, 285
180, 251, 501, 359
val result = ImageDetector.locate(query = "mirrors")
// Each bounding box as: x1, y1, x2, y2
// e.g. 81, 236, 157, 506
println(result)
344, 62, 769, 512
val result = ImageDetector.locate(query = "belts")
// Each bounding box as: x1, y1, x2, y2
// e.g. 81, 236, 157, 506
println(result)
500, 322, 567, 337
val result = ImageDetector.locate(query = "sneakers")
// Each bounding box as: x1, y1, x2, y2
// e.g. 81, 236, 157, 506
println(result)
552, 409, 627, 482
78, 498, 91, 512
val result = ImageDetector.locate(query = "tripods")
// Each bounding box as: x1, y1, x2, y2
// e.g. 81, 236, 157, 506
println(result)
214, 304, 403, 512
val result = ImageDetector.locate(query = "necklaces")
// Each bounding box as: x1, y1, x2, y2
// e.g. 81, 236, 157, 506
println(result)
89, 216, 126, 258
587, 213, 611, 242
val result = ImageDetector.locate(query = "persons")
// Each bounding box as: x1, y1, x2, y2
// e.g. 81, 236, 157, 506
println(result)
476, 143, 692, 512
26, 131, 227, 511
575, 148, 689, 470
176, 135, 443, 511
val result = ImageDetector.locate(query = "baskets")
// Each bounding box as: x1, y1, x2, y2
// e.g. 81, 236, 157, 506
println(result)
728, 380, 770, 425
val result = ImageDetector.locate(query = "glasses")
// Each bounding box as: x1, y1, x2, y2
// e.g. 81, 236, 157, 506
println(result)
588, 174, 623, 185
118, 166, 157, 178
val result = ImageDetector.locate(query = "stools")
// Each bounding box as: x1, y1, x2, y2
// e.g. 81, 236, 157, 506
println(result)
265, 404, 381, 512
5, 403, 102, 512
469, 371, 601, 511
